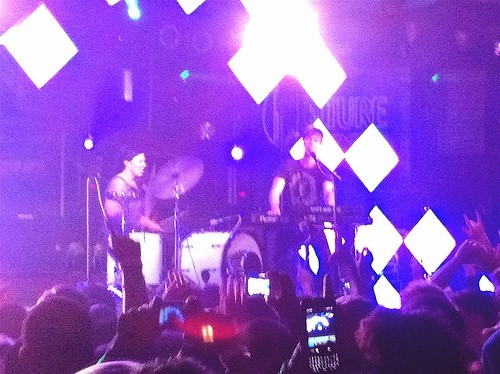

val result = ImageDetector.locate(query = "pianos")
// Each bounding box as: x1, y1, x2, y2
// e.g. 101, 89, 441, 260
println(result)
234, 207, 373, 227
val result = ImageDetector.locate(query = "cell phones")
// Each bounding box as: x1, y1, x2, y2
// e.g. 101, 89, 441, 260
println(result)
298, 296, 342, 374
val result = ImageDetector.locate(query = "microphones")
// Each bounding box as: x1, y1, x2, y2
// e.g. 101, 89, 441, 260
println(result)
209, 216, 238, 225
308, 149, 318, 162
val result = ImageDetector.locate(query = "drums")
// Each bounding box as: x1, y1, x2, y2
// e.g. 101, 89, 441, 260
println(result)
176, 229, 263, 290
127, 230, 172, 286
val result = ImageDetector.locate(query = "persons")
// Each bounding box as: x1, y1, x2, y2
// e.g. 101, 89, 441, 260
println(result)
103, 146, 164, 234
1, 208, 500, 374
267, 127, 348, 300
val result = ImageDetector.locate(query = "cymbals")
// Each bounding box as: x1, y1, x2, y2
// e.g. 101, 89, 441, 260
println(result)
149, 155, 203, 199
